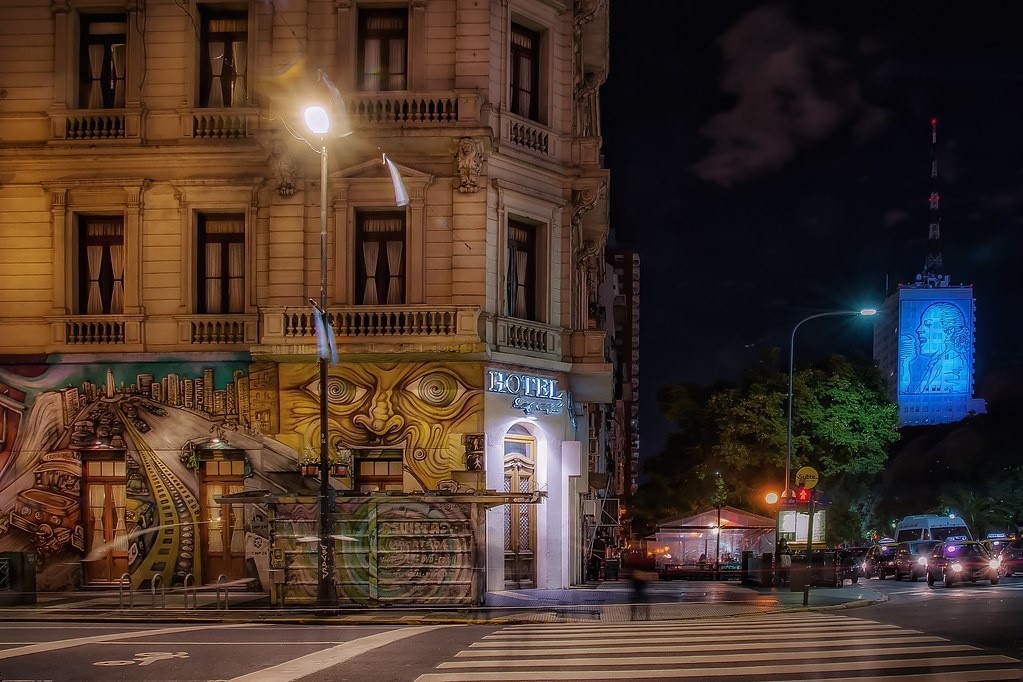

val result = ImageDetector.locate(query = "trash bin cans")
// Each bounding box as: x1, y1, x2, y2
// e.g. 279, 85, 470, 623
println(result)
0, 552, 37, 608
747, 553, 763, 581
790, 553, 809, 592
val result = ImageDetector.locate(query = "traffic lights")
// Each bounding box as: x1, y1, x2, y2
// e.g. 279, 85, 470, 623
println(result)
797, 489, 811, 501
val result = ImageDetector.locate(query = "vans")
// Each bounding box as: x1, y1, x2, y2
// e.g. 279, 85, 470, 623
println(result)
893, 514, 973, 543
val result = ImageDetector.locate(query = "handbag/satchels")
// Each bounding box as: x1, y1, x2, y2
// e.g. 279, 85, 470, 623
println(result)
781, 554, 791, 568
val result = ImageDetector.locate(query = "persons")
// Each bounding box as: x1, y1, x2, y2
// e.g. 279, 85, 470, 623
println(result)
775, 538, 792, 581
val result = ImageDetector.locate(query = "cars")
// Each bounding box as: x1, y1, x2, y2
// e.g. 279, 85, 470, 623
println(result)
998, 540, 1023, 578
845, 548, 870, 576
925, 536, 1000, 587
862, 538, 899, 581
979, 534, 1015, 557
833, 551, 858, 582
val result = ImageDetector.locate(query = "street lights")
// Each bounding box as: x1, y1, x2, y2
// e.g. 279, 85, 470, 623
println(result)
306, 106, 337, 605
785, 307, 877, 503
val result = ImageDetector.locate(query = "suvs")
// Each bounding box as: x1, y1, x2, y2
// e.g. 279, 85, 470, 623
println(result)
893, 540, 942, 581
